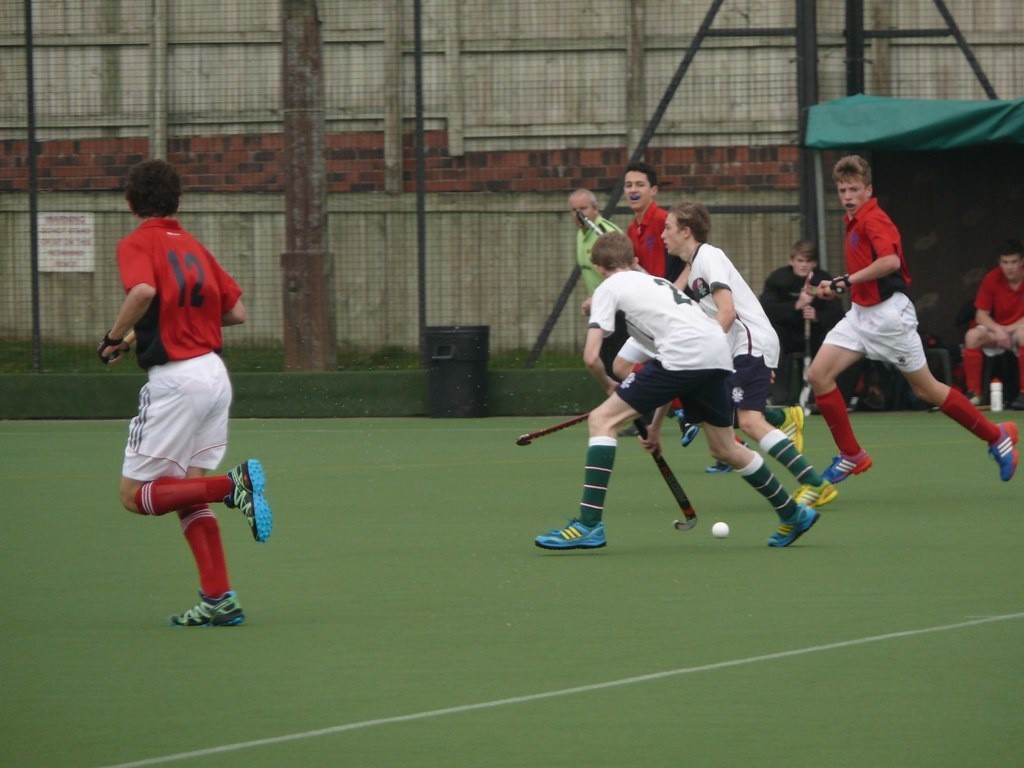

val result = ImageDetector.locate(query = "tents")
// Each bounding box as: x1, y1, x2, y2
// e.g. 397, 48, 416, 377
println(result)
806, 93, 1024, 276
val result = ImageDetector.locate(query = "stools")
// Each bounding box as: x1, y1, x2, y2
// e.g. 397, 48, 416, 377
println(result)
890, 346, 952, 408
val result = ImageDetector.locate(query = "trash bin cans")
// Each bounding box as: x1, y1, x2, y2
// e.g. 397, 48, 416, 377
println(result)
423, 325, 490, 418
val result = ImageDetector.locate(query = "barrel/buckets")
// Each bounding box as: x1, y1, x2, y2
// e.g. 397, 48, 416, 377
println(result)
424, 324, 489, 417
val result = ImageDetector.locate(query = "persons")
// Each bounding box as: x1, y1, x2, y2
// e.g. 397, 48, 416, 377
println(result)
580, 162, 750, 476
754, 236, 845, 406
531, 232, 821, 546
659, 200, 838, 508
96, 160, 273, 630
567, 189, 655, 437
962, 244, 1023, 411
806, 159, 1019, 485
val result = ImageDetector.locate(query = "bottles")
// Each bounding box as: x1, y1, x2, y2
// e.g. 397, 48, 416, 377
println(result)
989, 376, 1003, 412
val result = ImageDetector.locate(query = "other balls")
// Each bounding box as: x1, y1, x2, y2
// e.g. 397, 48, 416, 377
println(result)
712, 522, 730, 537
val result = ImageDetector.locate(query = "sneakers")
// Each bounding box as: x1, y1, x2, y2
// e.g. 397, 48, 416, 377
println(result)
680, 416, 697, 446
818, 449, 872, 485
778, 405, 804, 456
534, 518, 606, 551
987, 421, 1019, 482
706, 443, 748, 474
225, 459, 272, 543
172, 589, 245, 627
790, 478, 838, 508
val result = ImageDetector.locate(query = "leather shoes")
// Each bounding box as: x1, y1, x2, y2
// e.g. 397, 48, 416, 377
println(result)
765, 505, 818, 547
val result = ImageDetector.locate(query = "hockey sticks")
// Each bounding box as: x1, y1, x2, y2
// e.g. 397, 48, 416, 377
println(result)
799, 316, 812, 417
109, 330, 136, 359
811, 285, 843, 295
576, 211, 649, 274
632, 416, 697, 531
516, 412, 590, 446
846, 370, 865, 412
